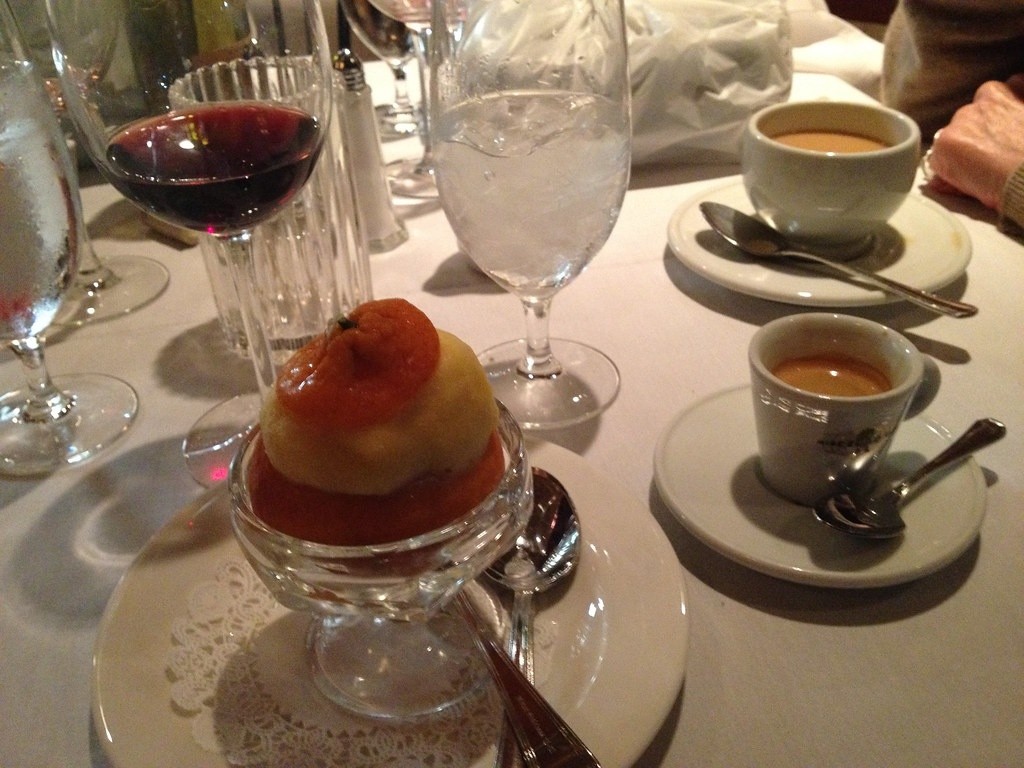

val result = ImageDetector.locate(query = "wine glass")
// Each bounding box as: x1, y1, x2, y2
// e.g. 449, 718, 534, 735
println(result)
0, 58, 139, 478
229, 397, 534, 723
40, 0, 334, 487
430, 1, 633, 429
366, 0, 439, 198
0, 0, 170, 329
340, 0, 419, 139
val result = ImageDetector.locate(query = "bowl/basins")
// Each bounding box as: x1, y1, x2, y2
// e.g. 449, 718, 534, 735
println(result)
741, 100, 922, 245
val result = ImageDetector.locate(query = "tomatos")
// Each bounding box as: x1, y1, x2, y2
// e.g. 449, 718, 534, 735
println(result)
275, 296, 440, 426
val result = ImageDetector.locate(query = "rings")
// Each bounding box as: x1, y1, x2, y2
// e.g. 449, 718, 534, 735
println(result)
934, 129, 944, 144
925, 150, 934, 161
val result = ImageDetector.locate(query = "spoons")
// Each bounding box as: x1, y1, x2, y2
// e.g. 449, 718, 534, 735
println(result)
699, 201, 978, 318
814, 418, 1006, 539
483, 466, 581, 768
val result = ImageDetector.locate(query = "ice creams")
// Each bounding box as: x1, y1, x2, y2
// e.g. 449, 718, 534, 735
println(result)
259, 327, 497, 498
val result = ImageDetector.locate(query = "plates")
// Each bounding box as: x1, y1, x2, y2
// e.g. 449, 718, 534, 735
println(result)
653, 383, 986, 587
94, 436, 688, 768
666, 180, 973, 307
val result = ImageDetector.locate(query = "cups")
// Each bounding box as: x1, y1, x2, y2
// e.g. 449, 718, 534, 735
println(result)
747, 311, 923, 507
168, 56, 376, 365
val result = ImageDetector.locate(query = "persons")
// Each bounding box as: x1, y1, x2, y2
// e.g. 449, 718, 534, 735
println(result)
881, 0, 1024, 233
